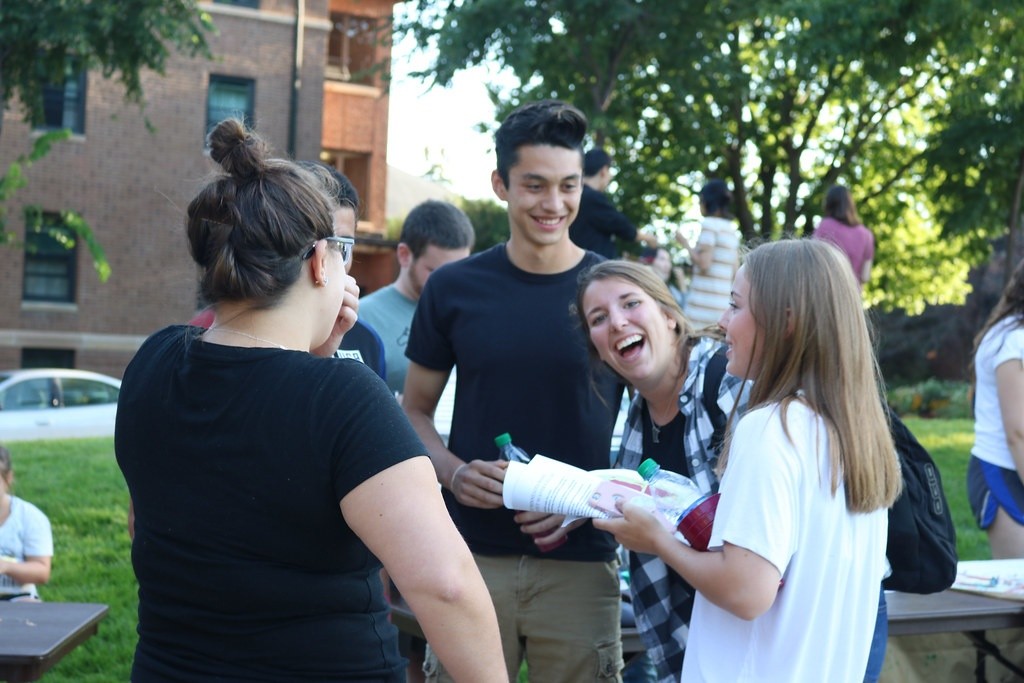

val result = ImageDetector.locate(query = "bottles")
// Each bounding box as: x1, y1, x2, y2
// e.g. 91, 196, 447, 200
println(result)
493, 433, 571, 552
636, 458, 708, 528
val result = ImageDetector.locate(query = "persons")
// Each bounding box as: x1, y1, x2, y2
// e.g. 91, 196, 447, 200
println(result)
402, 108, 633, 683
672, 180, 742, 328
965, 260, 1024, 559
115, 117, 508, 683
0, 443, 55, 602
638, 224, 679, 291
357, 199, 475, 398
811, 190, 873, 287
569, 147, 637, 260
581, 236, 907, 683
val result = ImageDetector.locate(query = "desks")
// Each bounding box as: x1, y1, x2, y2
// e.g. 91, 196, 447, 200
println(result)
887, 590, 1024, 683
0, 599, 109, 683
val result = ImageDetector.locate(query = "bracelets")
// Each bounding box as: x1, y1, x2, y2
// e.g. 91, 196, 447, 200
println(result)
450, 463, 468, 497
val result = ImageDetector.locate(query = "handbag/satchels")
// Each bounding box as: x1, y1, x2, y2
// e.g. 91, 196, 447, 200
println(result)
883, 405, 959, 595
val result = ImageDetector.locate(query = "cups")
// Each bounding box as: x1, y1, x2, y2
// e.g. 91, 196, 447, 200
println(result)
674, 493, 721, 553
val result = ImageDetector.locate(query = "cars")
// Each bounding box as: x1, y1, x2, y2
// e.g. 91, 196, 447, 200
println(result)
0, 365, 125, 445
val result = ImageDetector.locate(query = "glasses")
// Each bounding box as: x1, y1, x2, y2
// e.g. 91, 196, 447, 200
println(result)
302, 236, 355, 265
